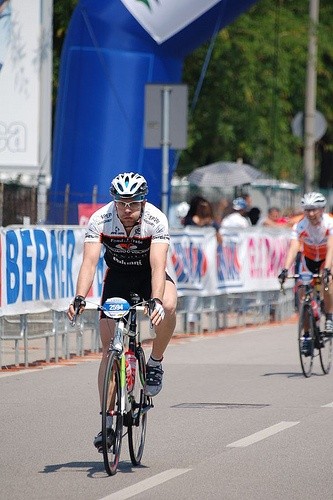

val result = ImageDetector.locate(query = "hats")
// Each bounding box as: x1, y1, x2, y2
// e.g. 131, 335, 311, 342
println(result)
233, 197, 248, 210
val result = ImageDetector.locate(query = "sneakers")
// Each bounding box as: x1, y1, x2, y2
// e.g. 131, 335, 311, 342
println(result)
324, 319, 333, 333
144, 362, 164, 396
93, 428, 115, 451
303, 340, 312, 355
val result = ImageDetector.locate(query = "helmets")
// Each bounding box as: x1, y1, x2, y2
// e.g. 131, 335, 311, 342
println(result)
109, 172, 150, 196
301, 192, 327, 209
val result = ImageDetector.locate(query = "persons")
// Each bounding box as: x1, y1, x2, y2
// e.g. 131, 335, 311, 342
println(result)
278, 192, 333, 354
68, 172, 178, 449
183, 193, 333, 229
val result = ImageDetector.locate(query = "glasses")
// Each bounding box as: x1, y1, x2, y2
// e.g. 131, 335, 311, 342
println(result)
114, 196, 145, 210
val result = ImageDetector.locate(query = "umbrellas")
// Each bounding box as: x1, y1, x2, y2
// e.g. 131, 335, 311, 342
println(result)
190, 159, 263, 188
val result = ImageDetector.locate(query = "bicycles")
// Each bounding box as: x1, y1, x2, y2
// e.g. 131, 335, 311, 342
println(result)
280, 268, 333, 379
71, 291, 155, 476
